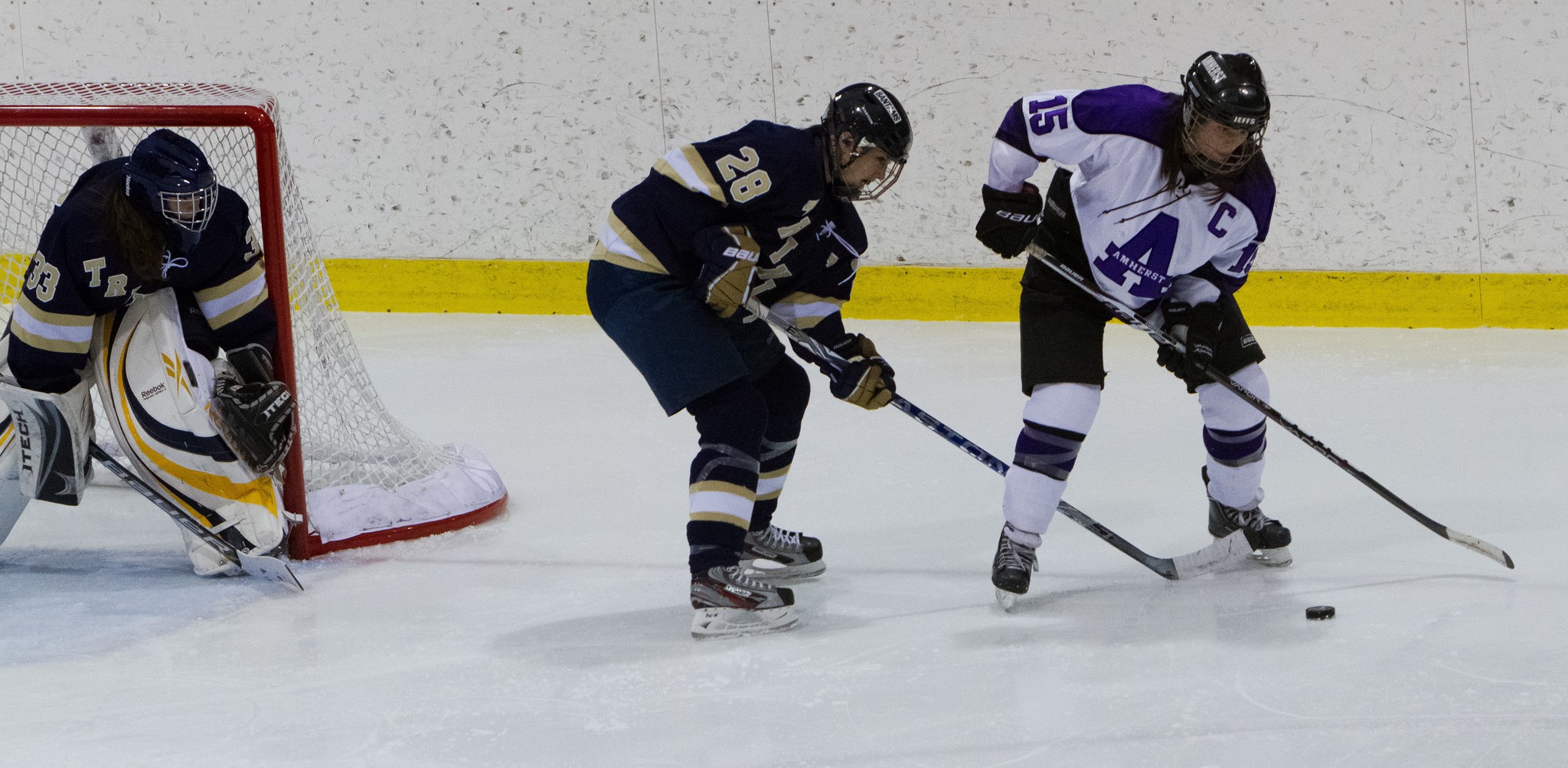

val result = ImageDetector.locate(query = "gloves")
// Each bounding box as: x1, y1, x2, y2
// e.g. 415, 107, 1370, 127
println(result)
702, 227, 763, 326
1156, 299, 1222, 380
830, 331, 896, 410
975, 181, 1043, 259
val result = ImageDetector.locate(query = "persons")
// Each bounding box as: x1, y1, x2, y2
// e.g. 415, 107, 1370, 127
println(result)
974, 50, 1293, 614
584, 84, 913, 638
0, 127, 296, 578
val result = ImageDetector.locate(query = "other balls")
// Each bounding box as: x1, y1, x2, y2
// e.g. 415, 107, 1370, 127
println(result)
1305, 605, 1336, 620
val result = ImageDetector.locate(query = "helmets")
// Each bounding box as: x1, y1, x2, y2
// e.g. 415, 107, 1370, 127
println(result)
823, 84, 913, 203
1180, 50, 1270, 178
125, 128, 217, 243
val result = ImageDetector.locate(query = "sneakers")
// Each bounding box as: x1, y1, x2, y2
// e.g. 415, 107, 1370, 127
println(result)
690, 565, 798, 636
990, 523, 1042, 612
1202, 463, 1293, 568
738, 526, 826, 582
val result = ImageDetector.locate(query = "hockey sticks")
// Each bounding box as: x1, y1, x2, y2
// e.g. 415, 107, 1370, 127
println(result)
1024, 241, 1521, 573
87, 439, 307, 593
746, 297, 1261, 584
